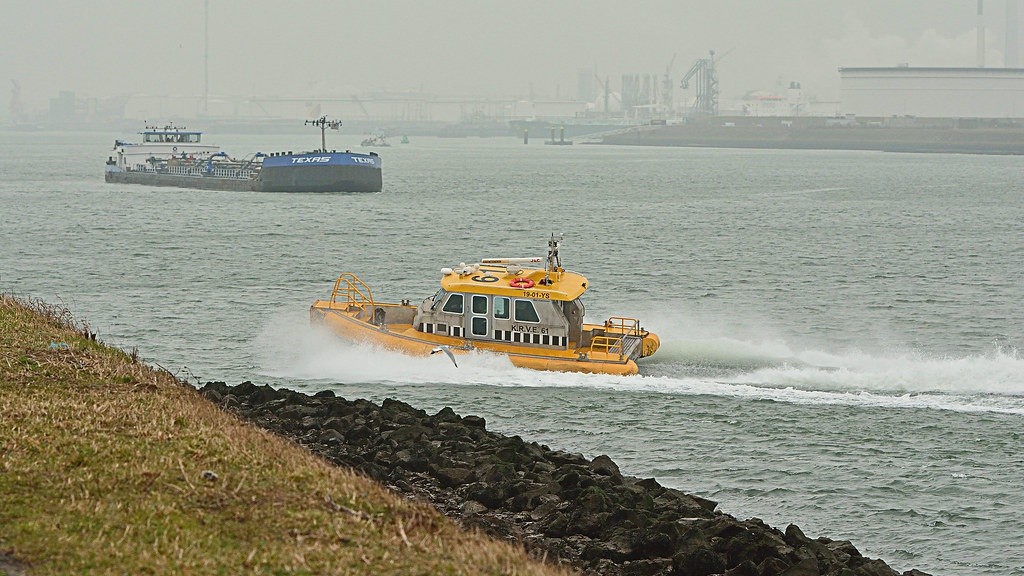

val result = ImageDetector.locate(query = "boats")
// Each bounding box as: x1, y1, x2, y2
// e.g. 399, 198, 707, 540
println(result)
309, 230, 661, 377
103, 112, 387, 194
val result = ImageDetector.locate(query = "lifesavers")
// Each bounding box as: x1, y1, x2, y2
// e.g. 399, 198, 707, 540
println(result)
509, 277, 535, 289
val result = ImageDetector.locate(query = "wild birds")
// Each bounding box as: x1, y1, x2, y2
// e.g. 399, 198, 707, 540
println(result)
430, 345, 458, 368
